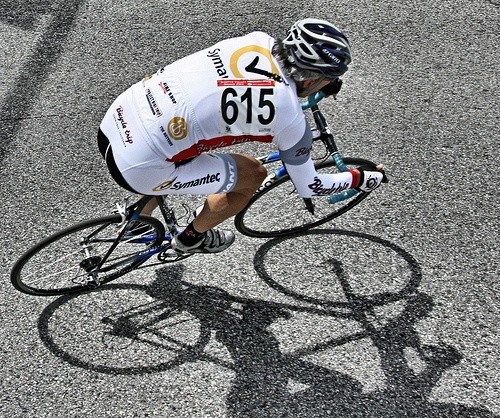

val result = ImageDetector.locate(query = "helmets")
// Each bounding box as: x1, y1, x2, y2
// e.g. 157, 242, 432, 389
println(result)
283, 19, 352, 78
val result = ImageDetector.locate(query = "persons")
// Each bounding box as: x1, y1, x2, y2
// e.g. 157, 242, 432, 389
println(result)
97, 18, 386, 254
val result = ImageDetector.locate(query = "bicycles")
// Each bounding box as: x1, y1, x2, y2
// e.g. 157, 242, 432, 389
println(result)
10, 90, 389, 297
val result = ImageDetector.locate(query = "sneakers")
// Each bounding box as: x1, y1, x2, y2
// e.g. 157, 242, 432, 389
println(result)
118, 220, 171, 240
171, 228, 235, 254
